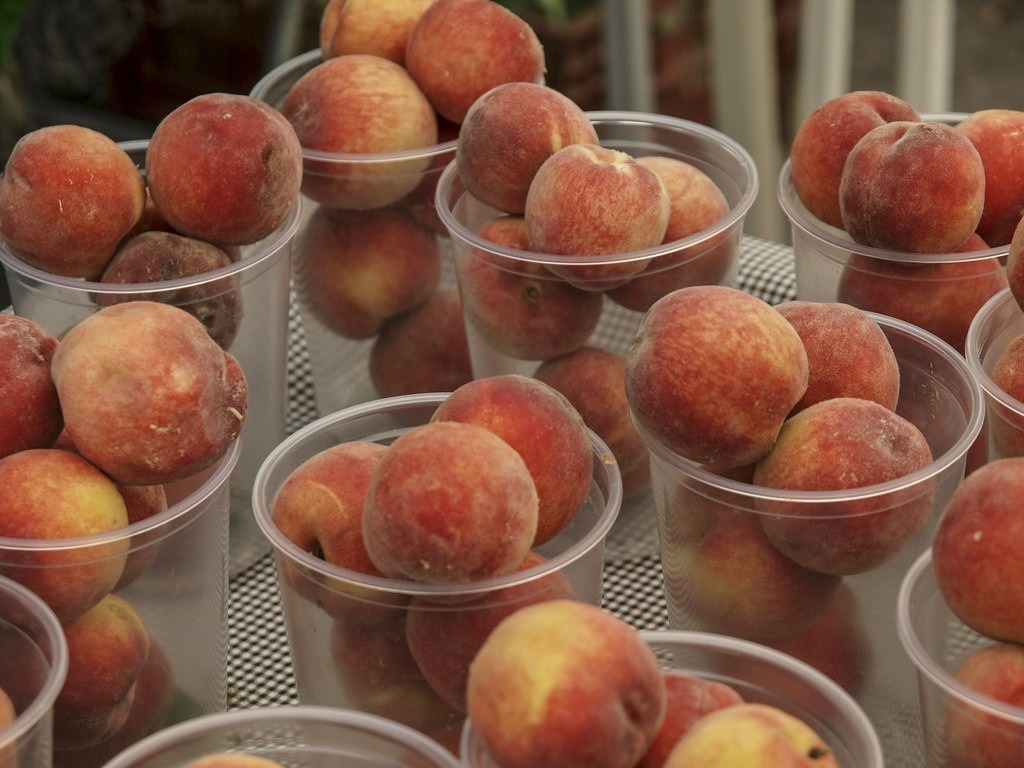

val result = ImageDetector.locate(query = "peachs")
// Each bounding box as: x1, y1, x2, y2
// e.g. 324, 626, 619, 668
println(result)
0, 0, 1024, 768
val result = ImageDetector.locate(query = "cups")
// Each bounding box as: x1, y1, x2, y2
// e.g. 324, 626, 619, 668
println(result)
776, 114, 1013, 477
965, 288, 1024, 477
250, 50, 545, 420
461, 631, 885, 767
896, 547, 1023, 768
250, 392, 624, 763
0, 576, 68, 767
0, 139, 302, 580
625, 313, 985, 764
103, 705, 460, 768
1, 426, 239, 767
434, 109, 760, 565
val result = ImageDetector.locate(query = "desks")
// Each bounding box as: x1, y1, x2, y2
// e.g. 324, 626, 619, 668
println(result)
0, 196, 1004, 768
